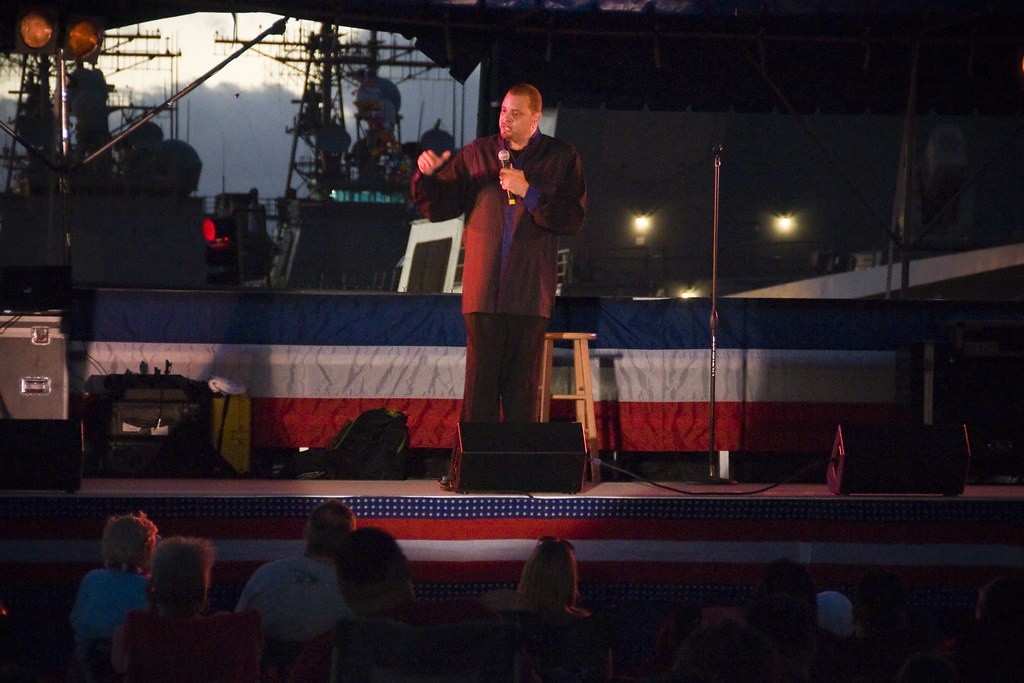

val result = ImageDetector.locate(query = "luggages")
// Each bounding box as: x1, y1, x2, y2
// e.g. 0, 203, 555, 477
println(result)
211, 389, 253, 480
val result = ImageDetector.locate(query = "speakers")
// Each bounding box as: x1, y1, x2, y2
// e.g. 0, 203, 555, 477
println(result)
825, 424, 970, 495
0, 418, 85, 491
450, 421, 589, 493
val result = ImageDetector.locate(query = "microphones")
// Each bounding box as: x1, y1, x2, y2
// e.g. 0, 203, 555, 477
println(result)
498, 150, 515, 205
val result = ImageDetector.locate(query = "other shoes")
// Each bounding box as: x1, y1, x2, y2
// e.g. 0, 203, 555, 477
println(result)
435, 471, 455, 494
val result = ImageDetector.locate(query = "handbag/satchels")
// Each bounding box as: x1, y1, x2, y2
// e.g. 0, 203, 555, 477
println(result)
327, 408, 412, 481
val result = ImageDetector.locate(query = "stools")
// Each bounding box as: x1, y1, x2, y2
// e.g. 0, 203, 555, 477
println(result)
533, 333, 601, 483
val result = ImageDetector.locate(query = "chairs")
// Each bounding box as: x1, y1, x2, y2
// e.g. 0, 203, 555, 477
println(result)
124, 610, 269, 683
334, 619, 516, 683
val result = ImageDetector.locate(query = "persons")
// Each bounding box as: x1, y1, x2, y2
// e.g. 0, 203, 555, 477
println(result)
0, 500, 1024, 683
410, 83, 583, 490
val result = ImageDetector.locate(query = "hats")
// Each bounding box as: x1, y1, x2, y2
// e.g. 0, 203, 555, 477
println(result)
813, 589, 860, 636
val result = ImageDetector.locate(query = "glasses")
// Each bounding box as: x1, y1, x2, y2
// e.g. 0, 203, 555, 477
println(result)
536, 534, 577, 556
